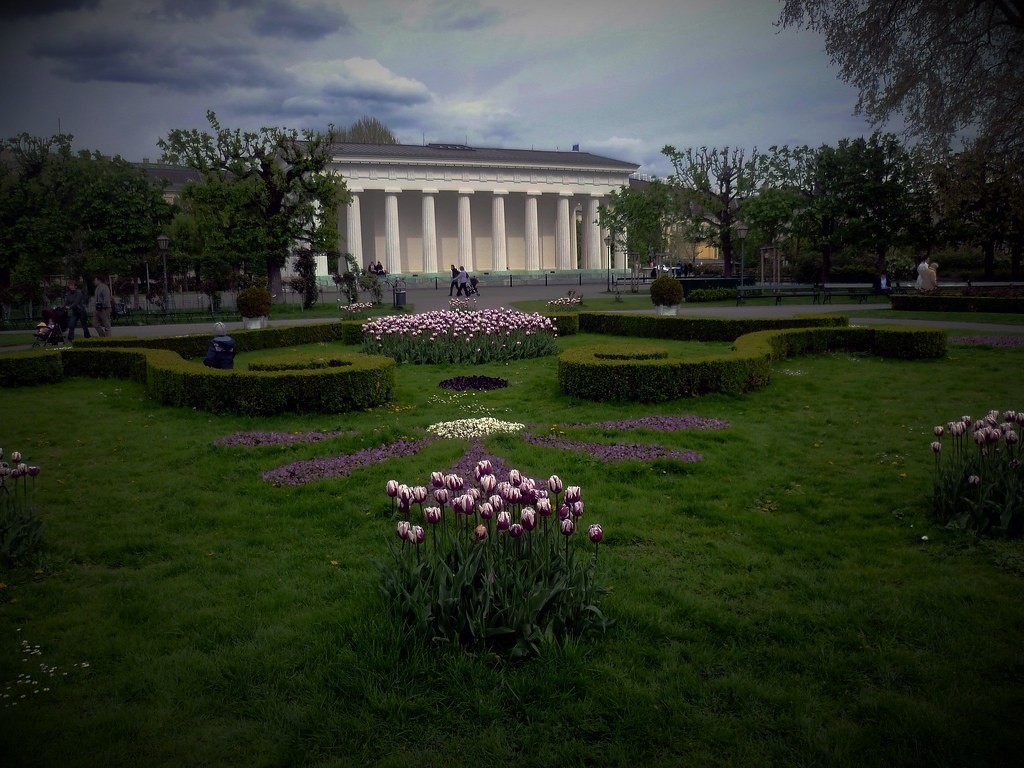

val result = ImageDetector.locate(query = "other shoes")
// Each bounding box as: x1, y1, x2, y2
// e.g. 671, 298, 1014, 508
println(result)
66, 339, 73, 346
466, 294, 469, 298
456, 293, 462, 296
448, 294, 452, 296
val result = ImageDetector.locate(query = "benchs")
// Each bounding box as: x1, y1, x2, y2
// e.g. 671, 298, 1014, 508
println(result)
613, 278, 657, 290
822, 283, 896, 304
971, 282, 1024, 288
778, 284, 820, 305
898, 282, 969, 294
735, 285, 780, 306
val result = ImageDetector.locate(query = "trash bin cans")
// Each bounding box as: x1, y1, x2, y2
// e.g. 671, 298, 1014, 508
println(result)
393, 287, 407, 309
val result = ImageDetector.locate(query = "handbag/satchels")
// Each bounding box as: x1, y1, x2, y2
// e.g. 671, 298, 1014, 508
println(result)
95, 302, 103, 311
466, 272, 469, 283
457, 270, 460, 276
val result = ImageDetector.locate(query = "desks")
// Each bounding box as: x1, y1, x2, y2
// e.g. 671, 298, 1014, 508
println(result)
0, 310, 240, 330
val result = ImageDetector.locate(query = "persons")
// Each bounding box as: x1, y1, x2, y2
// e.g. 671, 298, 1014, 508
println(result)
447, 264, 470, 297
914, 254, 939, 295
676, 260, 692, 277
41, 319, 55, 335
876, 273, 892, 302
368, 261, 385, 275
65, 281, 92, 342
651, 268, 656, 279
93, 276, 113, 338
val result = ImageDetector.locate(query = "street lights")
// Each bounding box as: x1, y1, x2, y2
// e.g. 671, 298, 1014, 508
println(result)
157, 230, 169, 316
735, 223, 750, 298
604, 234, 614, 293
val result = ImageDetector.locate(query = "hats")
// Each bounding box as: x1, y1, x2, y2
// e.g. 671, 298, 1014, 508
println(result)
67, 280, 78, 288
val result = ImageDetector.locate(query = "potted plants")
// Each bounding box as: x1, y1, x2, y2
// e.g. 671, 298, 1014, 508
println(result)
649, 275, 683, 316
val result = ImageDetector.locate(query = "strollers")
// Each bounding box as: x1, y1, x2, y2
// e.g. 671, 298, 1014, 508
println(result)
31, 306, 68, 349
